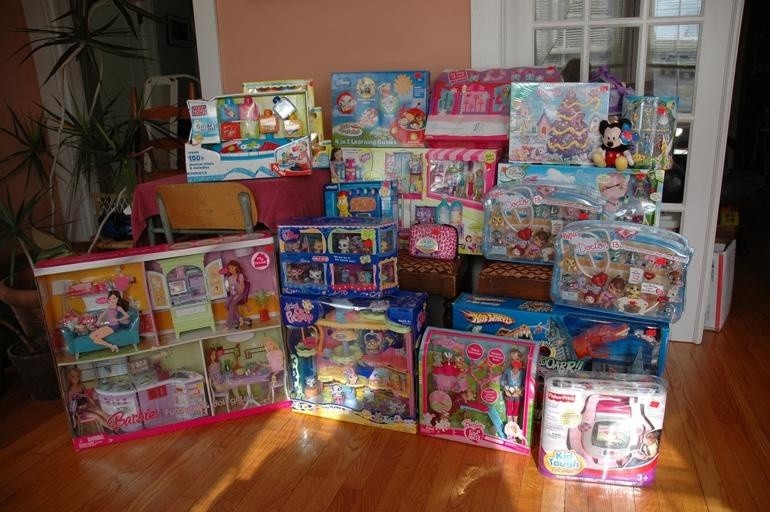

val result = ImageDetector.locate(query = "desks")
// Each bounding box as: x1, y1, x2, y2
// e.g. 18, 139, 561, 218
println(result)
127, 173, 332, 237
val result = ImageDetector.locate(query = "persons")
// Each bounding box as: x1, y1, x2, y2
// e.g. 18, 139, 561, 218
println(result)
33, 59, 690, 490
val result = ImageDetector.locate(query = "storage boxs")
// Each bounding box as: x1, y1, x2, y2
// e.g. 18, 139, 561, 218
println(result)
705, 239, 738, 333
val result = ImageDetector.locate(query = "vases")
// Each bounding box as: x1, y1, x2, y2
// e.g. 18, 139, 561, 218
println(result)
7, 338, 59, 405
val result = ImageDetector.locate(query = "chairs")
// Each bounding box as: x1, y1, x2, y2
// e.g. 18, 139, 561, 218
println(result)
155, 184, 261, 248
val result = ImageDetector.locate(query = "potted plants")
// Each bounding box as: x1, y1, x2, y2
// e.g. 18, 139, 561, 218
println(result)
2, 0, 181, 341
